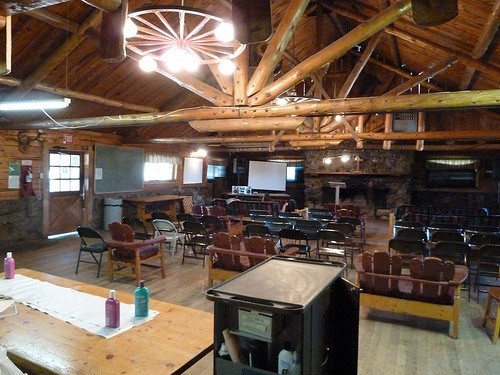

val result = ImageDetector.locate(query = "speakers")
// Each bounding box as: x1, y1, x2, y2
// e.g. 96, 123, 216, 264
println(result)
232, 185, 251, 196
233, 159, 245, 175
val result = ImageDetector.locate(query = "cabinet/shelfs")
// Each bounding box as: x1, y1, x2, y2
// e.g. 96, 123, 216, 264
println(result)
204, 253, 363, 375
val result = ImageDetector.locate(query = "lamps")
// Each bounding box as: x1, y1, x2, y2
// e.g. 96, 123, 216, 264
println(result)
0, 97, 71, 111
119, 5, 247, 75
323, 155, 360, 163
276, 96, 321, 105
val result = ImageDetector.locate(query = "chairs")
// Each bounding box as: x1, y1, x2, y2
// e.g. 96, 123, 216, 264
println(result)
76, 194, 499, 347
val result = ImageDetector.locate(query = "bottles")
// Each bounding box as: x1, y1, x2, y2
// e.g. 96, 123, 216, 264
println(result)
4, 252, 15, 279
222, 329, 246, 365
247, 341, 265, 370
218, 343, 230, 360
277, 340, 302, 375
134, 280, 149, 317
104, 290, 120, 328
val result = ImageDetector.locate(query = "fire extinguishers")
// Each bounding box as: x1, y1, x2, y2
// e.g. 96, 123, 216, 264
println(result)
24, 164, 33, 191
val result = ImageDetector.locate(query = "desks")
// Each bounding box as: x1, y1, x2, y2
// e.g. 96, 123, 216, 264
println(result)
0, 267, 216, 375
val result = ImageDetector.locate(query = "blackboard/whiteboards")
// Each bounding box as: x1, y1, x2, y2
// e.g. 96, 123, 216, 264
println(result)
93, 143, 146, 195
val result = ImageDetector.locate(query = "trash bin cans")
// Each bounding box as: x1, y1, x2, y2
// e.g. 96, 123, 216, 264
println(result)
103, 197, 123, 231
204, 254, 360, 375
